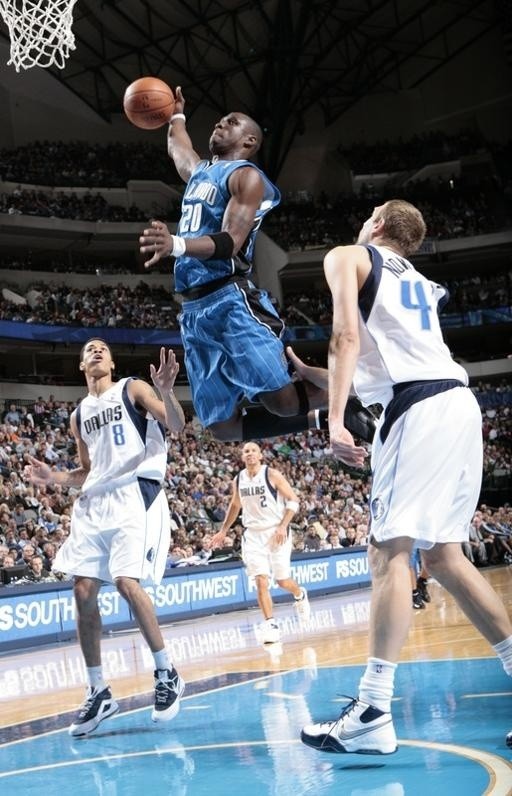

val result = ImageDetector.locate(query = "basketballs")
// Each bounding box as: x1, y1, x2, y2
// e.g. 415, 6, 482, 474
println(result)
124, 78, 176, 131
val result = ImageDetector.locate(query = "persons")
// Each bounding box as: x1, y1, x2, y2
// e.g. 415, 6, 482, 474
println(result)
335, 132, 507, 172
205, 443, 311, 644
24, 339, 186, 736
6, 188, 179, 225
2, 394, 76, 583
2, 279, 175, 332
141, 86, 297, 441
288, 198, 511, 754
254, 427, 372, 548
1, 139, 173, 186
434, 265, 510, 307
463, 377, 512, 567
408, 543, 431, 608
162, 409, 242, 564
282, 279, 331, 329
4, 245, 148, 276
279, 183, 507, 242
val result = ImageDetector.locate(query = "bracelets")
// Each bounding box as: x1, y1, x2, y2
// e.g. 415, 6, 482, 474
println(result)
169, 112, 185, 122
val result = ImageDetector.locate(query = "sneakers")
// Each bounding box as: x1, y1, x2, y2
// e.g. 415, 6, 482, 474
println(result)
151, 665, 186, 721
68, 685, 118, 737
300, 704, 398, 756
293, 588, 313, 622
411, 589, 425, 609
415, 579, 430, 603
262, 619, 281, 642
